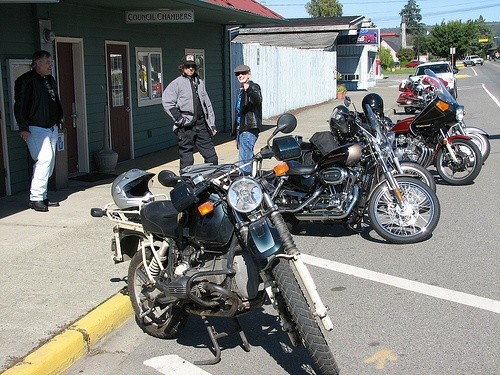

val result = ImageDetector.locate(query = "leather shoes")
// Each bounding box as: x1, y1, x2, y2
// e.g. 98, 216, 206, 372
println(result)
43, 199, 58, 207
28, 199, 49, 212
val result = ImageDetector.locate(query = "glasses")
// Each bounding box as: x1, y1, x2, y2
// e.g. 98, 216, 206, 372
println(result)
235, 72, 250, 77
184, 64, 196, 68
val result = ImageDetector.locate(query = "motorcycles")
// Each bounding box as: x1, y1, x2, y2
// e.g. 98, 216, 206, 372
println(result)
90, 111, 341, 375
239, 69, 491, 244
397, 74, 438, 114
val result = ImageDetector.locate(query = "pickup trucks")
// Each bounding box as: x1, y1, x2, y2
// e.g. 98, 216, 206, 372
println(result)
462, 54, 484, 66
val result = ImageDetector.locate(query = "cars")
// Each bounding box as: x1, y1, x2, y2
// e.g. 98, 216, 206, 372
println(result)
414, 61, 458, 101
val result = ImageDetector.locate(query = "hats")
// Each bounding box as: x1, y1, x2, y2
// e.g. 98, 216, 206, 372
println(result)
179, 54, 197, 67
233, 64, 251, 73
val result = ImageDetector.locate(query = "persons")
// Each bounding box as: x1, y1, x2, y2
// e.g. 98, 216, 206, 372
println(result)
234, 65, 262, 174
162, 54, 218, 171
14, 50, 63, 212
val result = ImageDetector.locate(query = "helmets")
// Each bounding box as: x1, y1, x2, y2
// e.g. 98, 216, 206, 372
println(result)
111, 169, 157, 210
362, 94, 383, 116
